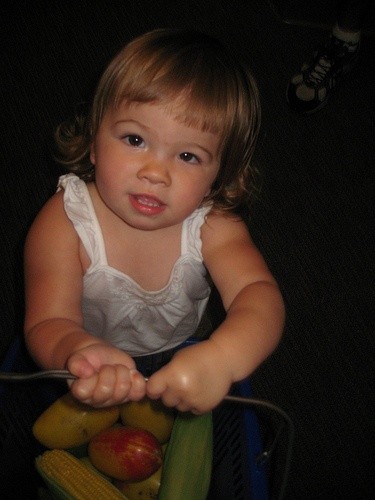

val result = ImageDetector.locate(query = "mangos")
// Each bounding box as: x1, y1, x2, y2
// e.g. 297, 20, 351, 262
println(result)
32, 390, 120, 449
88, 425, 162, 482
121, 396, 172, 440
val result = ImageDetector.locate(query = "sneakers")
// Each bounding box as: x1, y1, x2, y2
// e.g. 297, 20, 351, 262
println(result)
287, 36, 358, 114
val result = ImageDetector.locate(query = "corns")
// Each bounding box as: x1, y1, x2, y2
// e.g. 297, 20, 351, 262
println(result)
34, 448, 129, 500
158, 412, 214, 500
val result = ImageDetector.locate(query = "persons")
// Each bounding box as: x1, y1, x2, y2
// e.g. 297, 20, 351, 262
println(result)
288, 0, 367, 113
23, 25, 287, 417
